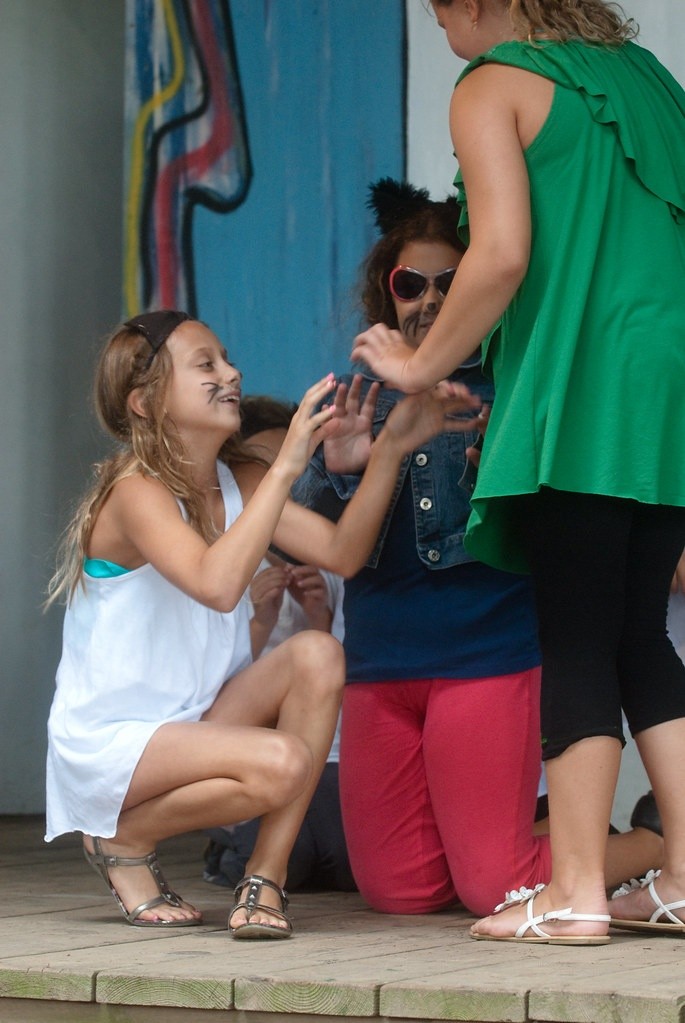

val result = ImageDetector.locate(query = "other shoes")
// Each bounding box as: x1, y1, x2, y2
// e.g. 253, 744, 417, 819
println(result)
608, 788, 664, 838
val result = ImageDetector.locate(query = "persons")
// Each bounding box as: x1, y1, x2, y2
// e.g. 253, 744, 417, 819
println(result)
207, 396, 346, 887
346, 0, 685, 945
293, 179, 664, 914
46, 311, 485, 936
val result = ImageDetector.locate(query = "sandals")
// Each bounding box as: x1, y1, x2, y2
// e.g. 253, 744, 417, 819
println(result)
469, 883, 612, 945
83, 835, 201, 928
228, 875, 292, 940
607, 870, 685, 932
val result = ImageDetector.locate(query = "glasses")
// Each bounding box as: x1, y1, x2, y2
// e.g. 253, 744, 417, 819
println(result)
389, 266, 457, 302
